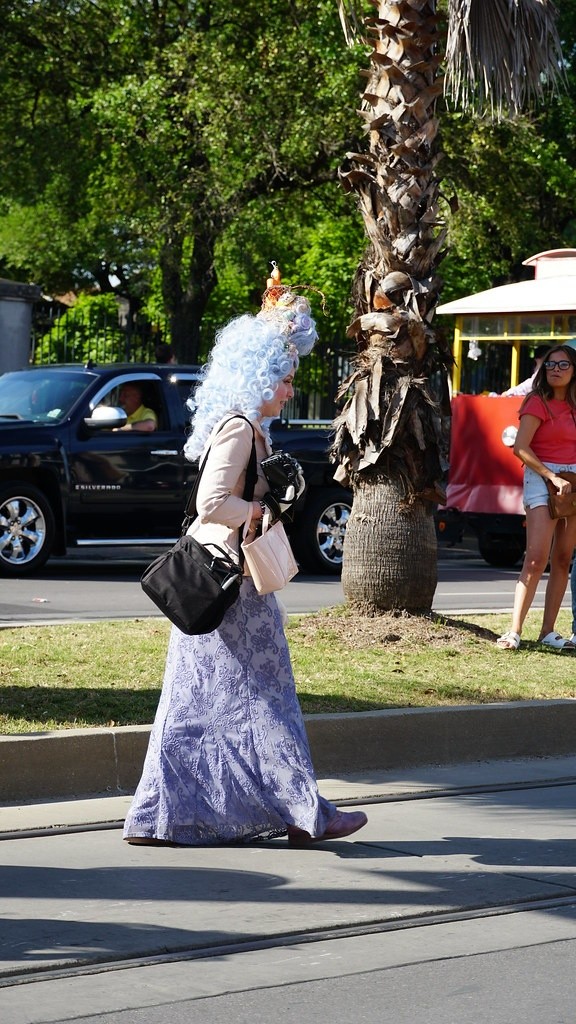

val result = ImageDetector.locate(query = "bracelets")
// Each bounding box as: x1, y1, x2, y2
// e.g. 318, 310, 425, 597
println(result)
256, 500, 265, 522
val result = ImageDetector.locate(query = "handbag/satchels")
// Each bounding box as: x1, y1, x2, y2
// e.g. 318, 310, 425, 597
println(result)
546, 472, 576, 520
241, 502, 299, 595
140, 537, 242, 635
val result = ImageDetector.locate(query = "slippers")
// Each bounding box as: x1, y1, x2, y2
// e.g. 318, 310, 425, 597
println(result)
497, 632, 520, 649
536, 632, 576, 649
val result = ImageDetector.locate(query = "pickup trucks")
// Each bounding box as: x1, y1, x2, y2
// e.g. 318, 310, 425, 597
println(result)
0, 360, 449, 578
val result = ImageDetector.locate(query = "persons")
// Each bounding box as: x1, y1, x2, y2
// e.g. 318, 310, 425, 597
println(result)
497, 344, 576, 651
153, 341, 178, 366
122, 318, 367, 844
112, 383, 156, 431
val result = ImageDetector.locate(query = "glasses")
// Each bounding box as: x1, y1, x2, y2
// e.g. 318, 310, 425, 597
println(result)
545, 360, 572, 370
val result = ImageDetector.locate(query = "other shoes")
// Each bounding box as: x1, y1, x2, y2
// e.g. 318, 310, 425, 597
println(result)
287, 811, 367, 847
